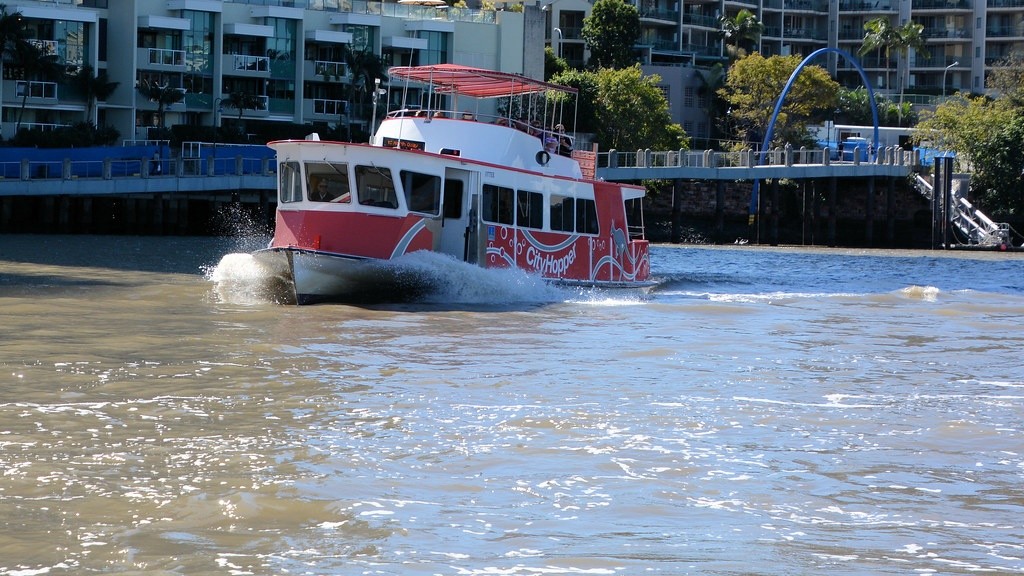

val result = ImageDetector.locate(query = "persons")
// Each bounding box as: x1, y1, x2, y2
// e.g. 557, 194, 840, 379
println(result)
463, 113, 473, 120
553, 124, 573, 158
310, 177, 334, 201
434, 111, 442, 117
150, 149, 160, 175
415, 111, 425, 117
498, 118, 552, 145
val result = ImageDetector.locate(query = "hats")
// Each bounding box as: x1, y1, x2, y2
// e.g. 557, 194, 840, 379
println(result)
497, 118, 507, 124
555, 124, 564, 129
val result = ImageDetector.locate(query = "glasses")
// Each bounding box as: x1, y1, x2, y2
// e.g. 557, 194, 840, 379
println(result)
533, 126, 540, 128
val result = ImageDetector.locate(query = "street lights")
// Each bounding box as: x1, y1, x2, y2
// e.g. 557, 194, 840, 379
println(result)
943, 61, 959, 102
369, 77, 387, 144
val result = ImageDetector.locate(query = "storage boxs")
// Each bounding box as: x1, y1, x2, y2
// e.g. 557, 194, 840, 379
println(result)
572, 150, 597, 179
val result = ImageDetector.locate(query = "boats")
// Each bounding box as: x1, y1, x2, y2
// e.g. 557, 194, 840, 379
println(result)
251, 65, 657, 306
